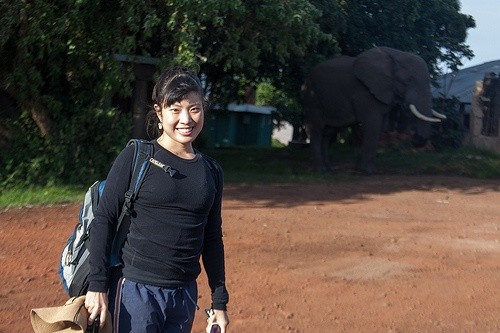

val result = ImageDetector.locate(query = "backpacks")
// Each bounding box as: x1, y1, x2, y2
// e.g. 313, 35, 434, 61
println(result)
59, 137, 222, 298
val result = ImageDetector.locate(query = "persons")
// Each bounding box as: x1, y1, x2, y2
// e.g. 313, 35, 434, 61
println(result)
80, 64, 231, 333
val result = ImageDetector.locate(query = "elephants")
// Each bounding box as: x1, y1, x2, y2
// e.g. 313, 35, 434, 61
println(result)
298, 46, 448, 177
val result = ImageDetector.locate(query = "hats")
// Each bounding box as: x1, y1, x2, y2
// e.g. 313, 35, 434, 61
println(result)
28, 296, 113, 333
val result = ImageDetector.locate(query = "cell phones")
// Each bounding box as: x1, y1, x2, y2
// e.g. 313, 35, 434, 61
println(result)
205, 309, 221, 333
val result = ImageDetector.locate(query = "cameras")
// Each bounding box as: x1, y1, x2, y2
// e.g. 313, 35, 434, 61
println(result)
87, 314, 99, 333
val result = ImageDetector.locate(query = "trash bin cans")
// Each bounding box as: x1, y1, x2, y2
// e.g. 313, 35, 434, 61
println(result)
287, 141, 309, 162
